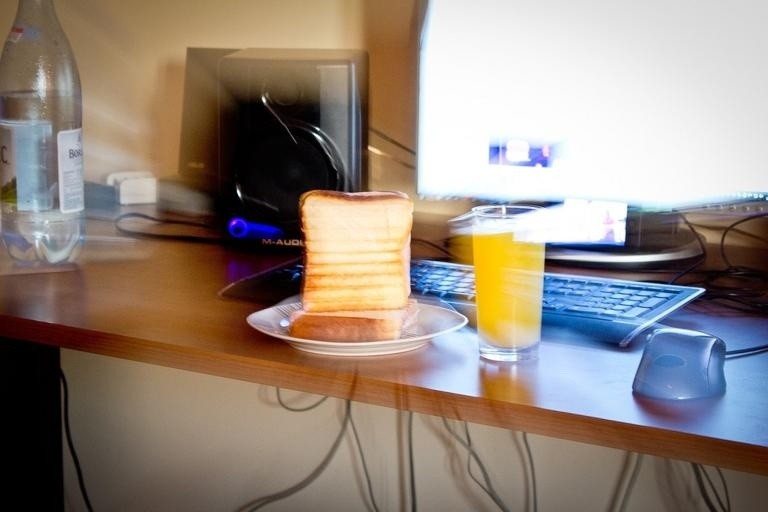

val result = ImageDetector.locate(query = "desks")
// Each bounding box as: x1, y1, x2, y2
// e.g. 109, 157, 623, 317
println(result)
3, 205, 768, 510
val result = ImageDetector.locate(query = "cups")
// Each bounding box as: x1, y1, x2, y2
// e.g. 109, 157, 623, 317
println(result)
471, 204, 548, 364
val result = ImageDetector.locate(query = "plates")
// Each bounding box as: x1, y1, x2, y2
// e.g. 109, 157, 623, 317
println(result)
245, 302, 470, 359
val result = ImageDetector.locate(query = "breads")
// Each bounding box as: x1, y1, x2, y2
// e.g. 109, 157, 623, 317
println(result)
287, 299, 419, 341
298, 188, 416, 314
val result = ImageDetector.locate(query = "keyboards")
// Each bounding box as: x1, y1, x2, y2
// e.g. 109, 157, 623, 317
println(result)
412, 254, 709, 350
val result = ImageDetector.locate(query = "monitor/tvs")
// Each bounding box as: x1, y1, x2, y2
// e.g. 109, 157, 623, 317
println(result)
413, 0, 768, 274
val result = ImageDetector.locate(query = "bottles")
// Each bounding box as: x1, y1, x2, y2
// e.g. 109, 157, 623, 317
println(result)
0, 0, 85, 266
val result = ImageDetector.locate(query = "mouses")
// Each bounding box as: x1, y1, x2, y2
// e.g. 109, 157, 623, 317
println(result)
631, 321, 726, 402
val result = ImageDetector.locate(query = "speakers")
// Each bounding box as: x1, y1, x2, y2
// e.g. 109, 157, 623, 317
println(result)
212, 47, 370, 306
